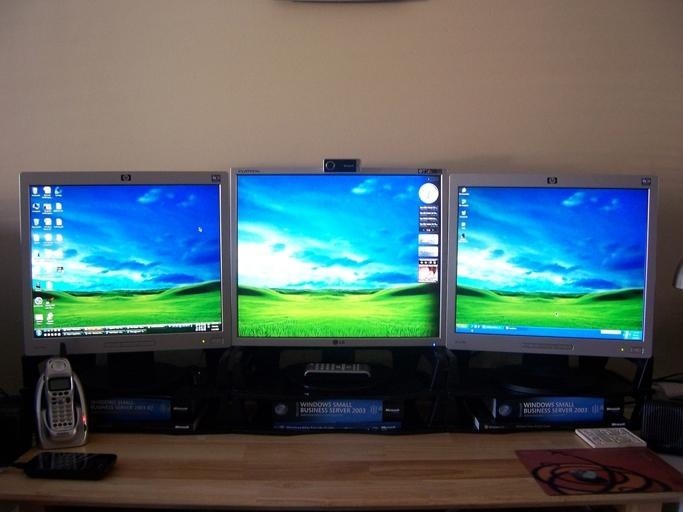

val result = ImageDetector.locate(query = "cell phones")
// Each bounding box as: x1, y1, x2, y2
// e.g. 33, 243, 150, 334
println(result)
1, 452, 116, 481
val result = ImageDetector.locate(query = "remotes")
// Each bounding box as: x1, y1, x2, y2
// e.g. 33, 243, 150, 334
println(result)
303, 361, 374, 379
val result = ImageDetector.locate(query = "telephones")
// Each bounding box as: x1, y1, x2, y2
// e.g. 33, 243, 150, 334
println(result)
35, 340, 89, 449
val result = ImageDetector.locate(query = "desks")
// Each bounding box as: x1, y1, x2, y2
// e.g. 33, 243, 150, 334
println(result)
1, 430, 682, 512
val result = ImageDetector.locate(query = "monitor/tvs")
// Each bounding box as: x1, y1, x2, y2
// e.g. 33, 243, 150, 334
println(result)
18, 170, 228, 400
449, 172, 658, 395
229, 165, 446, 393
